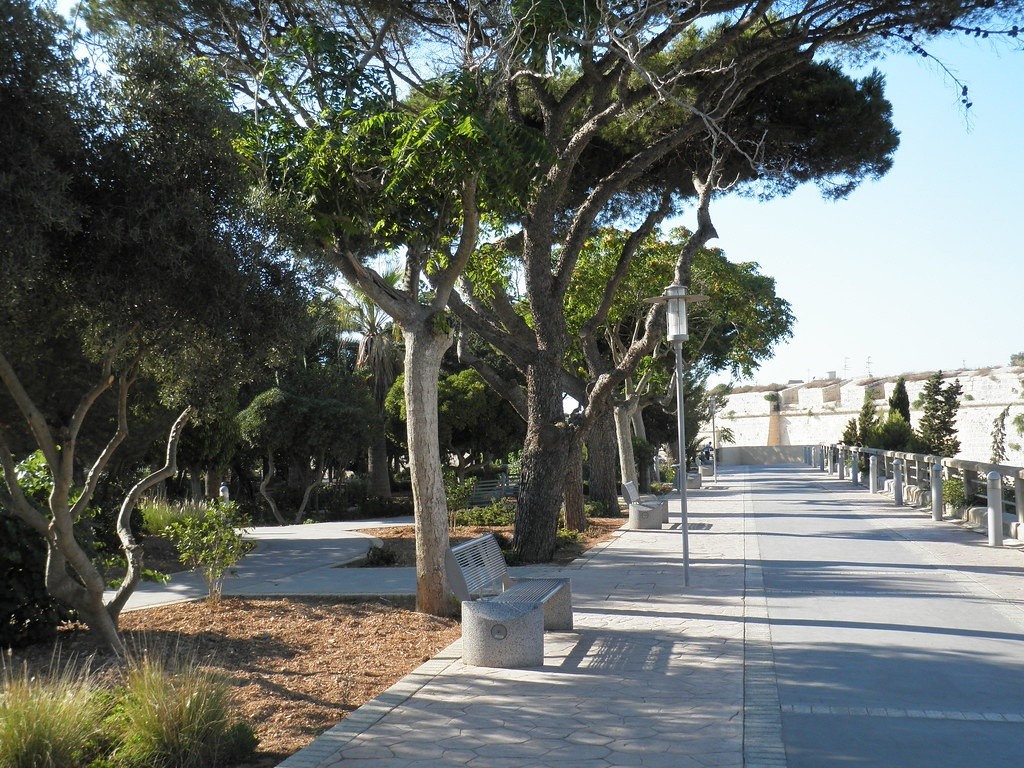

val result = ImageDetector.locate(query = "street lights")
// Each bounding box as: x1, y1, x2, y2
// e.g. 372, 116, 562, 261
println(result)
707, 396, 720, 484
642, 281, 712, 587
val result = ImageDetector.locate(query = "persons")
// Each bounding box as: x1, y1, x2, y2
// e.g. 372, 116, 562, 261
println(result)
703, 442, 713, 460
219, 482, 230, 505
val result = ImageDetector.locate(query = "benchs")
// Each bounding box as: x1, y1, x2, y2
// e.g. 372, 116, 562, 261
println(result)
696, 457, 714, 477
468, 472, 521, 508
445, 532, 573, 668
702, 454, 713, 465
621, 480, 669, 529
672, 464, 702, 489
